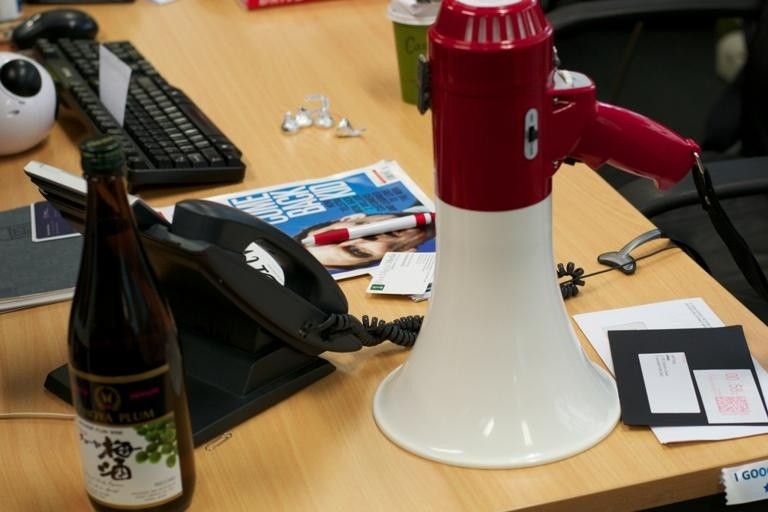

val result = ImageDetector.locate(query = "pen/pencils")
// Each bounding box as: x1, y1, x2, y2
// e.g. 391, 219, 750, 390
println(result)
301, 211, 435, 246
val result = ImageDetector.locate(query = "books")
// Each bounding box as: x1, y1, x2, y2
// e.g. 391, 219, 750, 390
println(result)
141, 152, 439, 285
1, 195, 84, 322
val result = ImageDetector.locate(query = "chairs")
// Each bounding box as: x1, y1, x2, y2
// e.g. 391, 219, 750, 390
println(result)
544, 1, 768, 324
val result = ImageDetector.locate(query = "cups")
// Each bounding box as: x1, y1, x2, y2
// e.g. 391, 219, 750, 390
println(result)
386, 0, 440, 105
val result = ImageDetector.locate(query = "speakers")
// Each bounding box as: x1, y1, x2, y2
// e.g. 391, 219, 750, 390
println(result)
0, 52, 58, 157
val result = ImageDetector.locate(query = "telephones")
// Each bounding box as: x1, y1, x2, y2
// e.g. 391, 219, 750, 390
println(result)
24, 158, 363, 451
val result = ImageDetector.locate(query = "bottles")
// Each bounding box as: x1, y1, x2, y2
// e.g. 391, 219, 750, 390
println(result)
67, 135, 197, 512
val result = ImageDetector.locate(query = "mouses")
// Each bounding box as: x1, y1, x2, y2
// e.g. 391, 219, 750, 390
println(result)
10, 8, 99, 49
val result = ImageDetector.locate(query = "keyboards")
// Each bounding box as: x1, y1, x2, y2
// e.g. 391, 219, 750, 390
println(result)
30, 38, 245, 188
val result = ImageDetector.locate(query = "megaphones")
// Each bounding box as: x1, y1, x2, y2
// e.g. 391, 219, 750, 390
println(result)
370, 0, 701, 470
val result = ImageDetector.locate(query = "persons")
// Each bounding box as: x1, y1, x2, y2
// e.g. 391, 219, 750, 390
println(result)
292, 201, 436, 271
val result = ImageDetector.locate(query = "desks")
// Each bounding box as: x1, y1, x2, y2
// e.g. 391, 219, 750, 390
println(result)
0, 0, 768, 512
